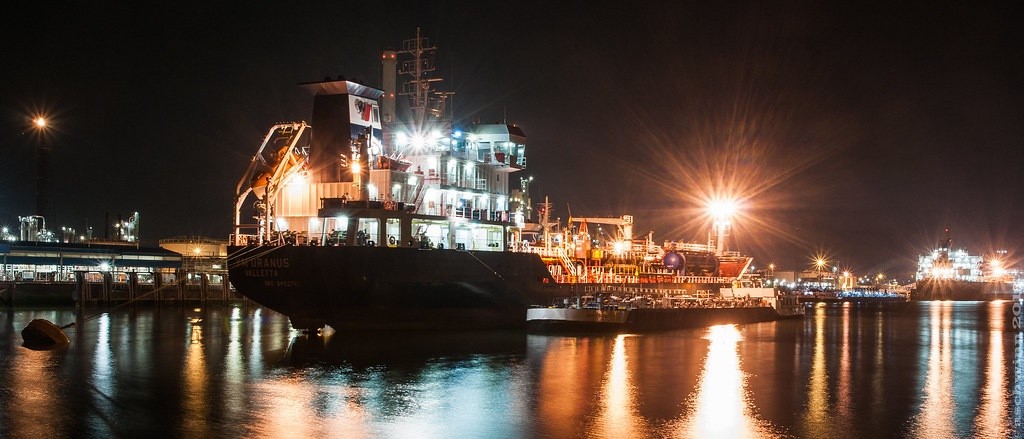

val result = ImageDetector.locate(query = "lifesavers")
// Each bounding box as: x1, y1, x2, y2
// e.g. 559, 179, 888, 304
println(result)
389, 236, 396, 245
385, 201, 394, 211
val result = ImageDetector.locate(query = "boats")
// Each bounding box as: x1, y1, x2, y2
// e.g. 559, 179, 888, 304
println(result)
524, 247, 629, 327
225, 26, 558, 331
